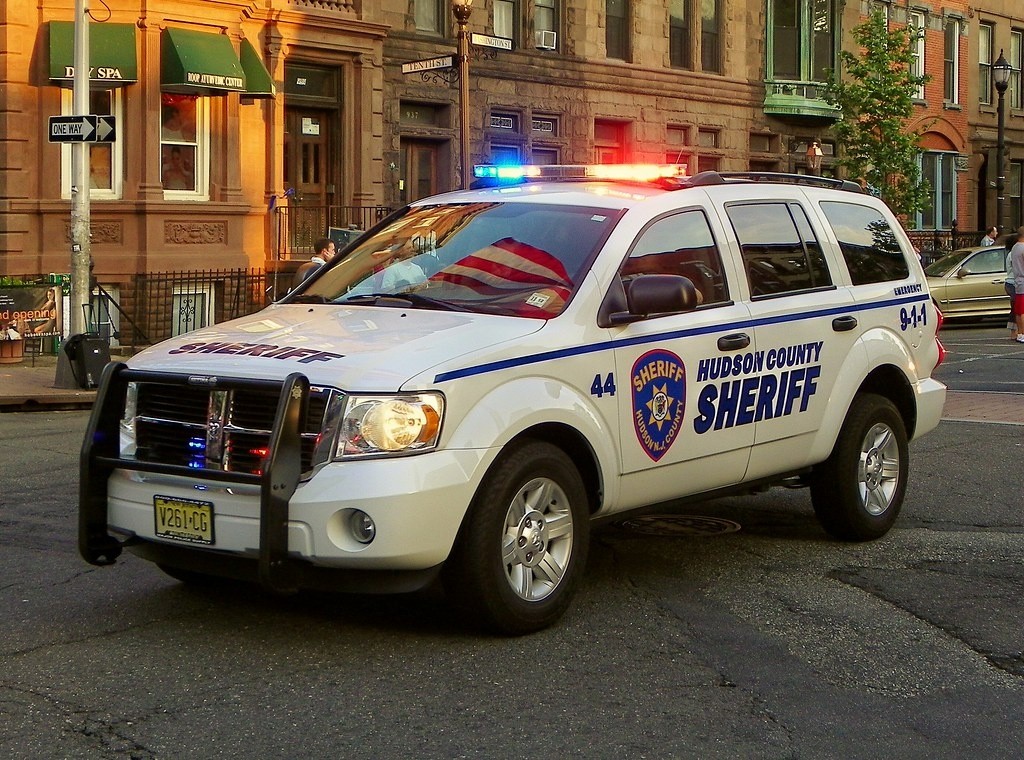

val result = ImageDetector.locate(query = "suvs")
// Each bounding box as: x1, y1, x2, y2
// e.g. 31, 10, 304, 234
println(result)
77, 164, 948, 638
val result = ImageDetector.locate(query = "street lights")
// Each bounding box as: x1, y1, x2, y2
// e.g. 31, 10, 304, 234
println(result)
452, 0, 474, 190
992, 48, 1013, 238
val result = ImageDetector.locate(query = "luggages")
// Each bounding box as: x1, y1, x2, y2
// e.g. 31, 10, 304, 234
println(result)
79, 304, 111, 391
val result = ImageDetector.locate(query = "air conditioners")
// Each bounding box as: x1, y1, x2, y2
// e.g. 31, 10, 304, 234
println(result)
535, 31, 556, 49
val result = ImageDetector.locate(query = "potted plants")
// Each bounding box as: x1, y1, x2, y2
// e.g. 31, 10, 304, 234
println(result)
0, 328, 25, 363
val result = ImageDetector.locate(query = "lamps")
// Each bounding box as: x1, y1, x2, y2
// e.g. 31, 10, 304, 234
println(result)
788, 136, 824, 170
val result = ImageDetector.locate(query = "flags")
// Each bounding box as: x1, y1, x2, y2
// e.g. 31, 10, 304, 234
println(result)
408, 236, 575, 319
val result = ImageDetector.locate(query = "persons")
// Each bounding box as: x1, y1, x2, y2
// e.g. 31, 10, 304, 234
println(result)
309, 238, 335, 266
1004, 235, 1019, 340
912, 240, 926, 270
34, 288, 56, 334
979, 226, 998, 247
1011, 225, 1024, 343
369, 239, 429, 293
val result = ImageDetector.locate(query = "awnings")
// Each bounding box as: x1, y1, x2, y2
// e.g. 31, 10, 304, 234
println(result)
239, 36, 277, 100
49, 20, 138, 82
159, 26, 246, 94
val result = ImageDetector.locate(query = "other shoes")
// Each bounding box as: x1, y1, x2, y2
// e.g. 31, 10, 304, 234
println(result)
1010, 330, 1013, 339
1013, 330, 1018, 339
1016, 334, 1022, 341
1020, 335, 1024, 342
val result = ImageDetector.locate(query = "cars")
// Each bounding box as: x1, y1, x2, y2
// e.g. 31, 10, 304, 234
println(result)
923, 245, 1011, 324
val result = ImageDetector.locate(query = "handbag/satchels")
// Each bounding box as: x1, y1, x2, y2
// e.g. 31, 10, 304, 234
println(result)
64, 332, 99, 382
